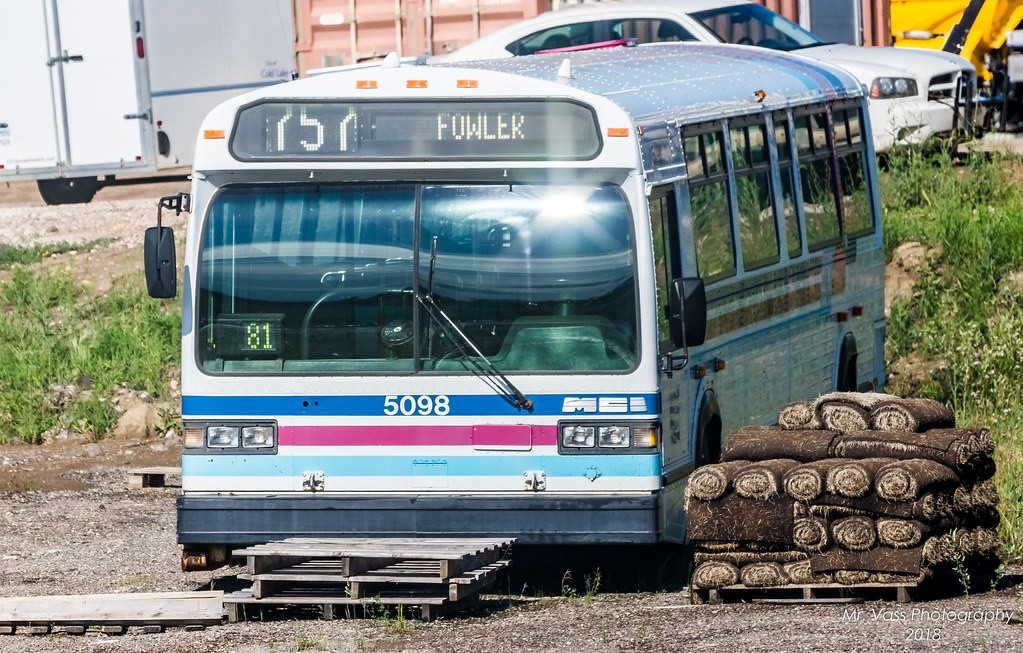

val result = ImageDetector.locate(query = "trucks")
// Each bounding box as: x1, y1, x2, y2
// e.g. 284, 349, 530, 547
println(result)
0, 2, 295, 206
292, 0, 895, 81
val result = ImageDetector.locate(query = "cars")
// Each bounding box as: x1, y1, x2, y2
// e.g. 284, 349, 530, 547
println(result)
307, 0, 982, 159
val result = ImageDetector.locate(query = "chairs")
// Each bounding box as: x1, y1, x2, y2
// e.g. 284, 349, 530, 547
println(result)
658, 22, 678, 42
541, 34, 571, 50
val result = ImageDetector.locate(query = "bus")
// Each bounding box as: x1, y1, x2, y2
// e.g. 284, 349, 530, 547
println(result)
142, 32, 889, 586
